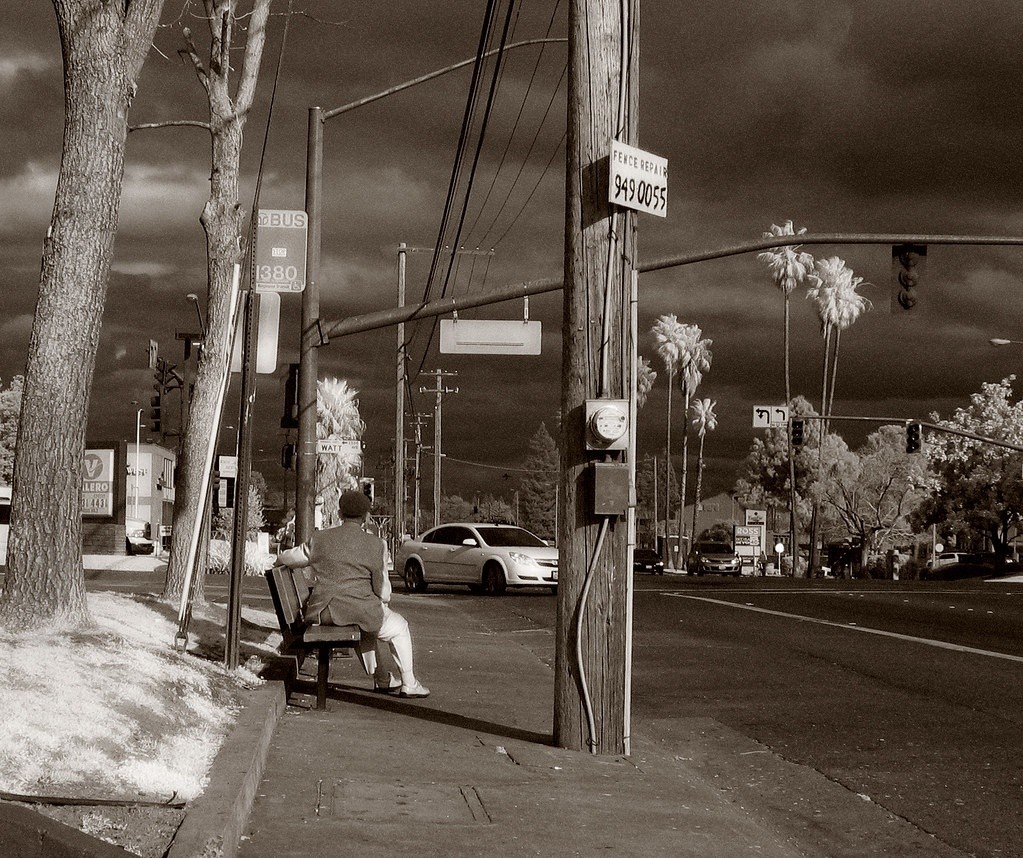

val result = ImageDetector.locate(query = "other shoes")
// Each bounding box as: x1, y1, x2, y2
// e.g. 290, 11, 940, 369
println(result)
400, 683, 430, 697
377, 672, 403, 692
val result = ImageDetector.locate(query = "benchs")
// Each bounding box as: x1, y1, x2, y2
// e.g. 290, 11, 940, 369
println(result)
266, 563, 363, 713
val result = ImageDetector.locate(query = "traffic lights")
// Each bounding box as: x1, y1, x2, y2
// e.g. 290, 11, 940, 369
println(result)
791, 420, 803, 446
906, 424, 922, 454
149, 356, 178, 433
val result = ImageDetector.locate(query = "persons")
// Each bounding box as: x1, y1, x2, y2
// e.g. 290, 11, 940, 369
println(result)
273, 491, 431, 699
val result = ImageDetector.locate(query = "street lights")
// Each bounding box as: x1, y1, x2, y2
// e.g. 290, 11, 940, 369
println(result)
187, 294, 205, 336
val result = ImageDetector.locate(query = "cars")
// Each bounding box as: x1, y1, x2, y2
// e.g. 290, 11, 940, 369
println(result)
395, 523, 559, 596
633, 548, 664, 576
926, 553, 967, 572
686, 541, 740, 578
126, 516, 154, 556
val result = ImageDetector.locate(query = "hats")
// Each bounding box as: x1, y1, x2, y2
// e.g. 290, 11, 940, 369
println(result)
340, 490, 370, 517
